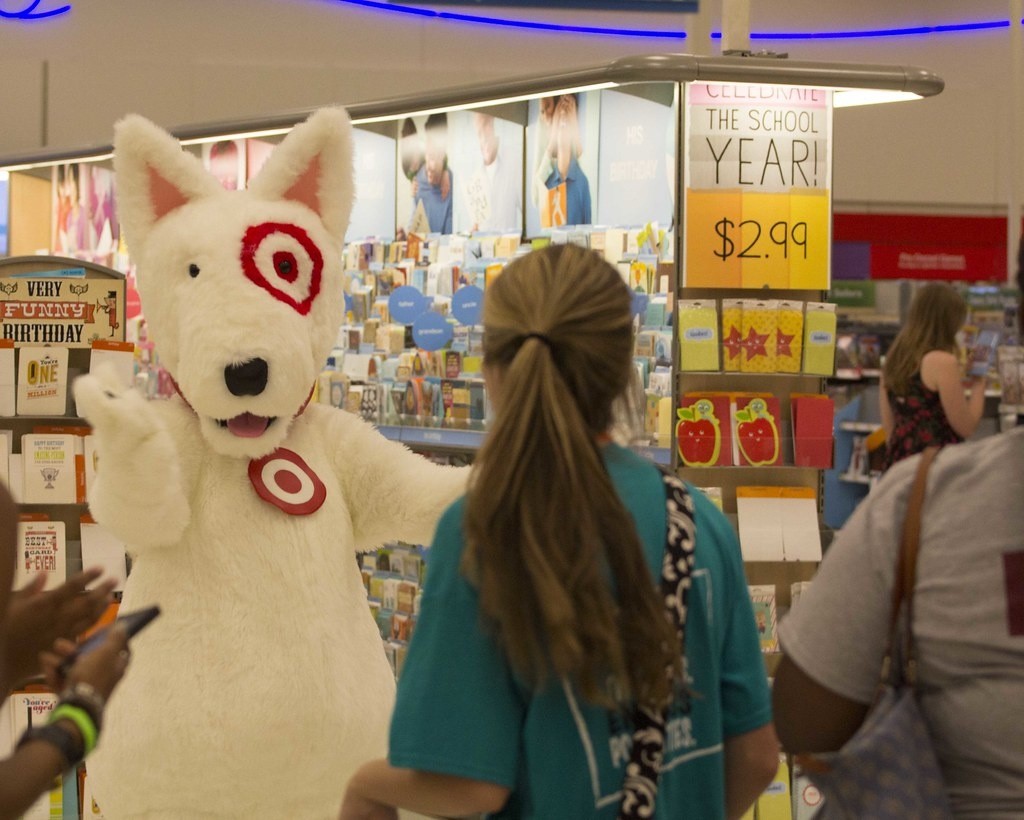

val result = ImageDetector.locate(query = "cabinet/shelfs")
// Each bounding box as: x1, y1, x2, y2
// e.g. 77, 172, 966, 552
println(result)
0, 53, 1024, 820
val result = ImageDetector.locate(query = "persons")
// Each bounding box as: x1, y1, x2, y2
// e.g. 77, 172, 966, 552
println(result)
339, 243, 780, 818
401, 112, 454, 235
879, 283, 990, 477
770, 427, 1023, 820
538, 93, 591, 227
0, 480, 132, 820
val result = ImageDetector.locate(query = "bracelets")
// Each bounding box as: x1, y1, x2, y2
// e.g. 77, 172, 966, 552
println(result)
45, 682, 103, 759
15, 726, 84, 767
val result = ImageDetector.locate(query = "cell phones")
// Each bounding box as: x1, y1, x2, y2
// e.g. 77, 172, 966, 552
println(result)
65, 606, 160, 665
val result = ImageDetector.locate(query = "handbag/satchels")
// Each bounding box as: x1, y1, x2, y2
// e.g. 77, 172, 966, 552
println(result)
788, 446, 953, 820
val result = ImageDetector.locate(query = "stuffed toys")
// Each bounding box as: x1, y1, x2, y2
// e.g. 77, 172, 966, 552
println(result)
74, 104, 479, 820
